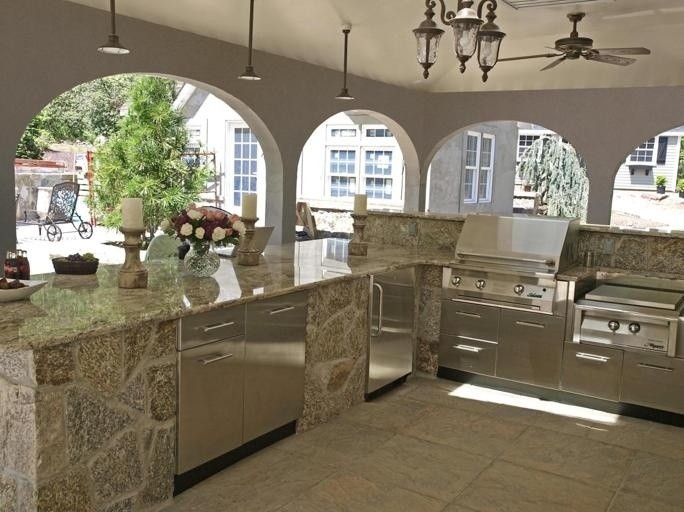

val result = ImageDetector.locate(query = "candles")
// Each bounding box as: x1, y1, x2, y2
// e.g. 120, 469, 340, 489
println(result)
353, 194, 367, 215
120, 197, 143, 229
241, 193, 257, 219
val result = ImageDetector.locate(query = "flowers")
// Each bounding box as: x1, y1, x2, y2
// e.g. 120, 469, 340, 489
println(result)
159, 208, 247, 257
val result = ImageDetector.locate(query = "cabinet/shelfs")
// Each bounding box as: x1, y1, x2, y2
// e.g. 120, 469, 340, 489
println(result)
364, 266, 415, 403
173, 289, 307, 495
436, 300, 565, 405
560, 341, 684, 427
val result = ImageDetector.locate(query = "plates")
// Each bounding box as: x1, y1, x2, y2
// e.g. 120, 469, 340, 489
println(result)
0, 279, 48, 303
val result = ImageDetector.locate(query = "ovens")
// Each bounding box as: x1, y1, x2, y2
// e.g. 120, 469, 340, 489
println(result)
574, 301, 677, 358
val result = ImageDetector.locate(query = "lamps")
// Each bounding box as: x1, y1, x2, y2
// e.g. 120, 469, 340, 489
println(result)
411, 0, 507, 85
236, 1, 263, 83
333, 23, 355, 101
95, 1, 130, 56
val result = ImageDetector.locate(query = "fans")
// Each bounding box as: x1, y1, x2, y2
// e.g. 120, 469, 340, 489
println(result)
496, 12, 649, 72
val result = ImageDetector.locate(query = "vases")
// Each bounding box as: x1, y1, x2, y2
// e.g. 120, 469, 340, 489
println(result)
182, 240, 220, 277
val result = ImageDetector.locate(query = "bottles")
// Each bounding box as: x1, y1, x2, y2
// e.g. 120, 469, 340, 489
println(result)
584, 249, 594, 268
5, 247, 31, 278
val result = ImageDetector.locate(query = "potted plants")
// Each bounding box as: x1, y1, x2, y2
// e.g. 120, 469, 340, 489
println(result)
655, 175, 667, 195
676, 179, 684, 198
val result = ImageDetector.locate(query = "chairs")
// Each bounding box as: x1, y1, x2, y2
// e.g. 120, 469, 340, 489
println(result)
15, 181, 92, 241
